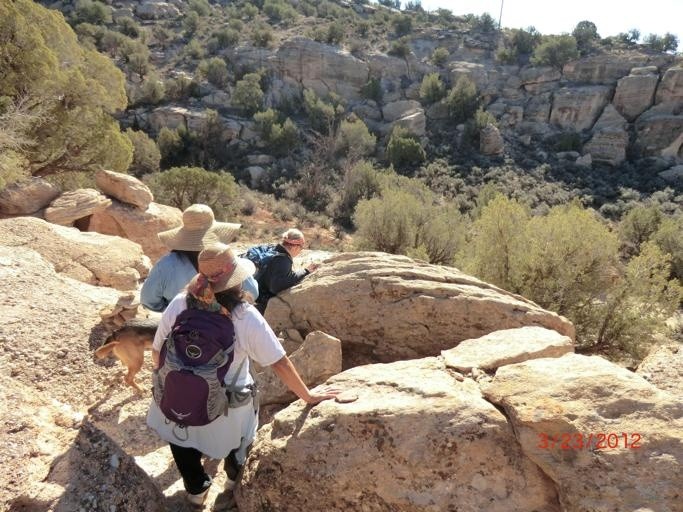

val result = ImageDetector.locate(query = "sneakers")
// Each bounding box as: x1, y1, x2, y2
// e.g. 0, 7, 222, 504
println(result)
223, 475, 236, 492
187, 489, 209, 506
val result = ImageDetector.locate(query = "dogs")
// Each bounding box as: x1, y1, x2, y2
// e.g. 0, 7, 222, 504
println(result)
95, 322, 160, 397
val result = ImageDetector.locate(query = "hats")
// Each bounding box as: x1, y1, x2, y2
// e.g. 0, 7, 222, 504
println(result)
185, 241, 257, 299
157, 203, 243, 252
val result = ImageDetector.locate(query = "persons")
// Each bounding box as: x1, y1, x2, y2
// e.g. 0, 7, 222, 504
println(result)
145, 240, 342, 506
236, 228, 321, 317
140, 203, 259, 312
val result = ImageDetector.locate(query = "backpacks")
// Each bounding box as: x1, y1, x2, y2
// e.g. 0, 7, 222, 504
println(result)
151, 292, 245, 426
